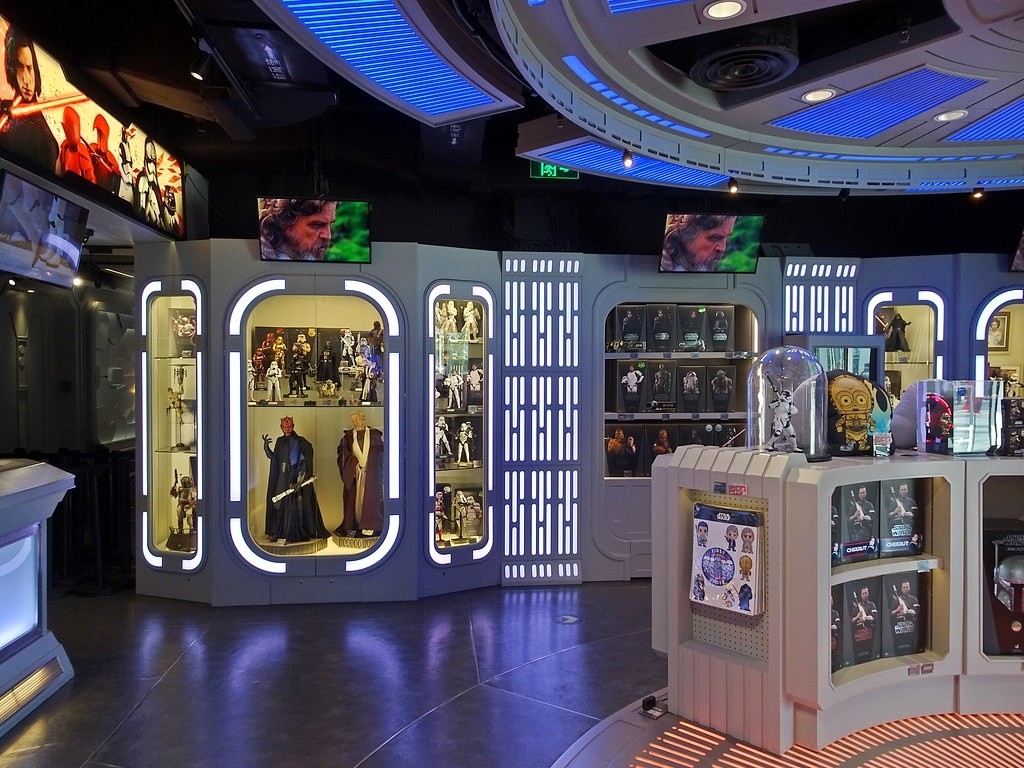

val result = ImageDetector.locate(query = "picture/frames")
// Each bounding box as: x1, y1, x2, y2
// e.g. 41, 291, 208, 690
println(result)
988, 310, 1013, 355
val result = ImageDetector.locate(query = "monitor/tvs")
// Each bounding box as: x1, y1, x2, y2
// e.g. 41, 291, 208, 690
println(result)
0, 168, 90, 291
1008, 230, 1024, 272
256, 196, 372, 264
658, 212, 767, 275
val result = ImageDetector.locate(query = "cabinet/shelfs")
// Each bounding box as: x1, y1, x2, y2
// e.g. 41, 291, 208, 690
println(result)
436, 338, 485, 546
156, 354, 198, 456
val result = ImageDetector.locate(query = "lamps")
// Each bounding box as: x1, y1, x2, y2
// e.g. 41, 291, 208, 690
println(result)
557, 113, 564, 128
622, 148, 633, 168
972, 188, 985, 200
838, 188, 851, 201
727, 176, 739, 195
190, 51, 214, 81
8, 274, 23, 286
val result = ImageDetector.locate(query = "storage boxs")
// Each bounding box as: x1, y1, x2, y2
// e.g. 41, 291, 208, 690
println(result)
605, 304, 744, 478
831, 379, 1024, 675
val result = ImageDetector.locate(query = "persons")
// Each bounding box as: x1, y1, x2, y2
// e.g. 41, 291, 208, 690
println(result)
435, 417, 476, 463
452, 491, 482, 536
435, 300, 482, 341
939, 413, 953, 435
444, 363, 484, 410
833, 484, 923, 650
434, 491, 448, 541
875, 314, 912, 353
168, 383, 189, 424
172, 311, 196, 346
258, 199, 341, 261
606, 308, 734, 464
0, 25, 60, 174
990, 369, 1023, 397
765, 389, 802, 452
334, 411, 384, 536
170, 476, 197, 535
262, 416, 332, 544
662, 215, 742, 272
248, 321, 384, 401
